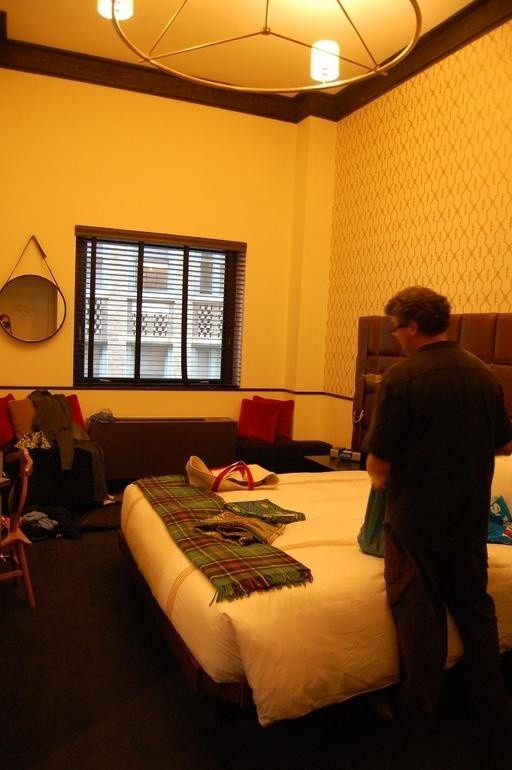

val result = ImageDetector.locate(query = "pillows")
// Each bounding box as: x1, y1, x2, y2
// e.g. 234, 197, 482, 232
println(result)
237, 394, 296, 444
1, 392, 86, 445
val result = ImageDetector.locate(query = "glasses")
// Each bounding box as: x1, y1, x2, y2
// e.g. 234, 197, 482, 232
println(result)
387, 323, 403, 336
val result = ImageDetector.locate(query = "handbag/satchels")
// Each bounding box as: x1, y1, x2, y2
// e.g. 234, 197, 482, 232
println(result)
185, 455, 279, 492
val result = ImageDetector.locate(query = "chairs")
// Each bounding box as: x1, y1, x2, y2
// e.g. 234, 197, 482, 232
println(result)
1, 446, 38, 610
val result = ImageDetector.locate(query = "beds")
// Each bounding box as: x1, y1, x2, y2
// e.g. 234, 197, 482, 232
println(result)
117, 455, 510, 732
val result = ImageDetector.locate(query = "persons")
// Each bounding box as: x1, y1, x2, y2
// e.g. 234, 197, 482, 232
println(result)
361, 284, 511, 743
